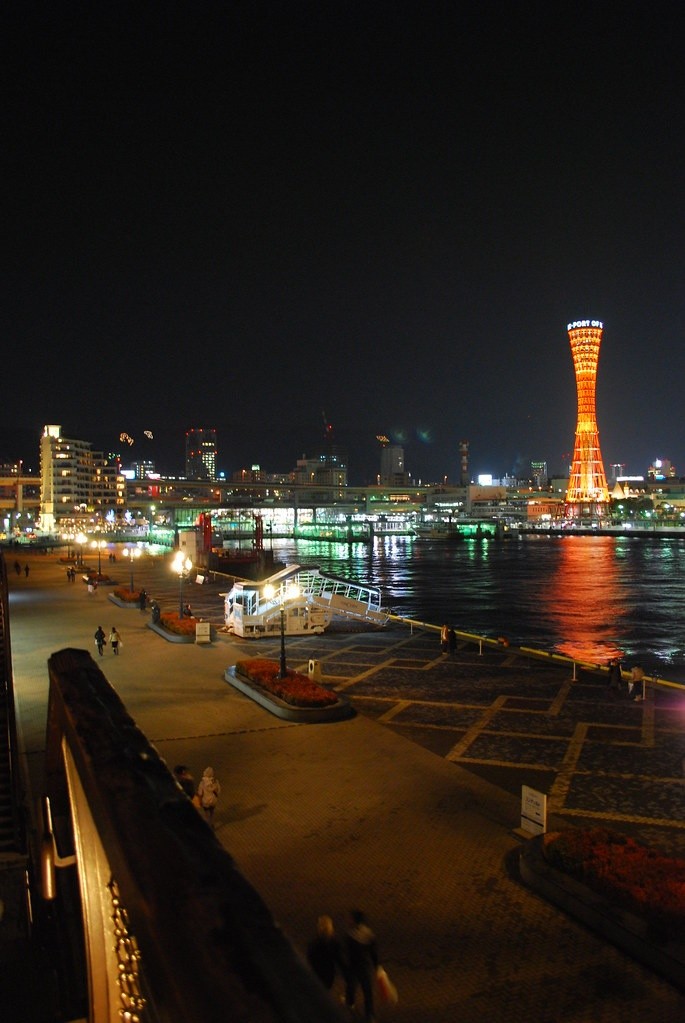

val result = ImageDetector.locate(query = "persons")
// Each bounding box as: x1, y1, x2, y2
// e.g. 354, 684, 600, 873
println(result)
95, 625, 105, 657
448, 626, 458, 655
198, 767, 221, 822
184, 604, 193, 616
308, 916, 349, 991
67, 568, 71, 582
87, 576, 94, 593
631, 663, 644, 702
109, 553, 116, 563
15, 562, 21, 575
108, 626, 121, 656
608, 659, 623, 690
440, 624, 449, 654
71, 567, 76, 583
140, 589, 147, 611
340, 910, 379, 1023
174, 765, 196, 800
24, 564, 29, 577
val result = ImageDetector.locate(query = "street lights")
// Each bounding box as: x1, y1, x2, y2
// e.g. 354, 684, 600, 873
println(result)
76, 532, 88, 567
170, 549, 193, 619
262, 583, 301, 680
91, 539, 108, 576
121, 547, 141, 594
62, 533, 74, 560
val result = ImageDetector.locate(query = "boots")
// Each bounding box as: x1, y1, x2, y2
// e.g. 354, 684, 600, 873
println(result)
634, 696, 641, 702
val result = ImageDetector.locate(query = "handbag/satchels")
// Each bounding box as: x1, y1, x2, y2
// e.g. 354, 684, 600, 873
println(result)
120, 642, 123, 647
192, 796, 201, 809
95, 639, 97, 644
376, 969, 398, 1007
